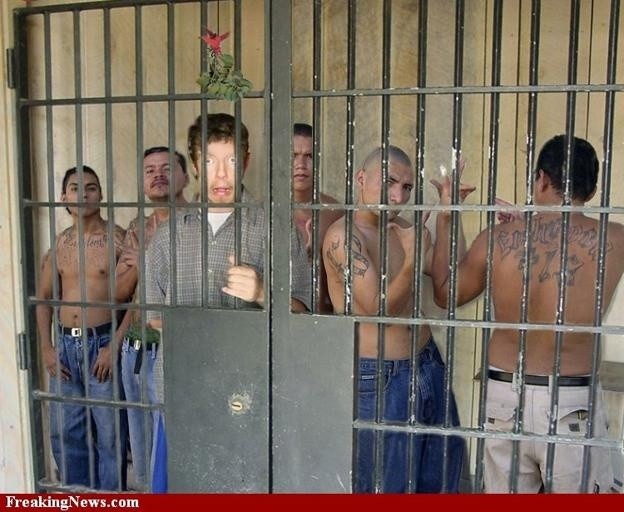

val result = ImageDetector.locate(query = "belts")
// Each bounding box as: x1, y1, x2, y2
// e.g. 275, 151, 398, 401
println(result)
128, 338, 157, 374
57, 321, 111, 338
472, 366, 600, 387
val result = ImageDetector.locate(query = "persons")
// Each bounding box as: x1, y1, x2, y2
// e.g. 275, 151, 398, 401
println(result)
115, 146, 192, 497
292, 123, 345, 315
136, 113, 314, 494
321, 145, 476, 494
433, 134, 624, 494
36, 166, 128, 492
